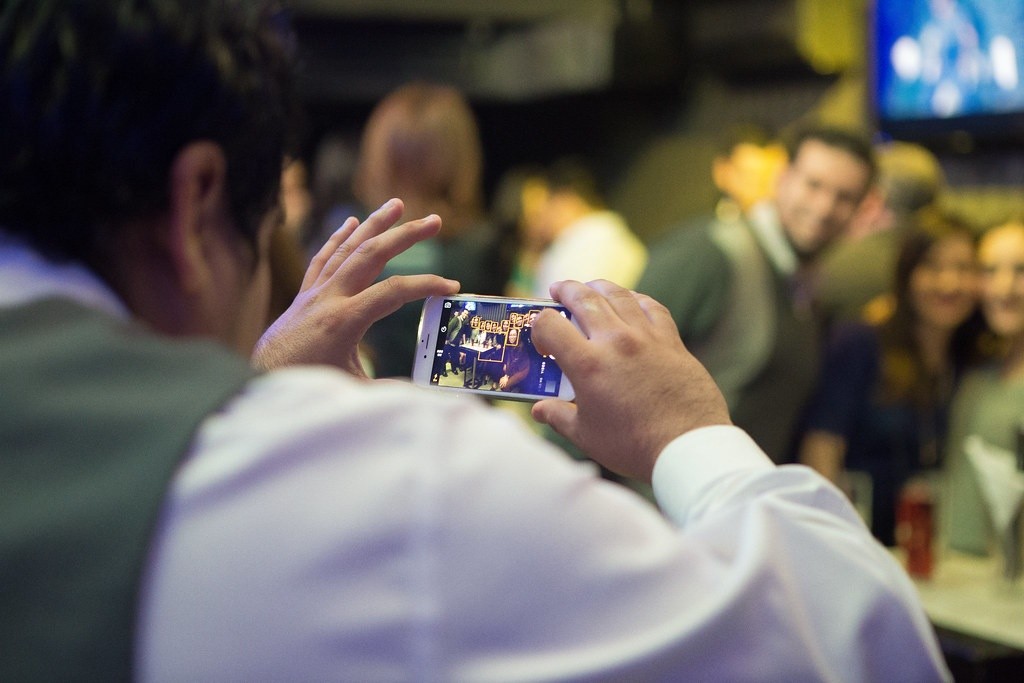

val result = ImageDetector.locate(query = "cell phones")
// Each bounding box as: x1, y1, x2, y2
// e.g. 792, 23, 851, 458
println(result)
413, 291, 589, 401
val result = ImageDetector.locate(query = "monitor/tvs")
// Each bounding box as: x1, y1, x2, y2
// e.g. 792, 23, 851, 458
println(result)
872, 0, 1024, 144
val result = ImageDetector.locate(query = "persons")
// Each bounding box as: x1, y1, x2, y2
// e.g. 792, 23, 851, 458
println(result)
519, 160, 649, 406
514, 118, 1024, 585
0, 0, 951, 682
273, 78, 508, 380
443, 307, 544, 394
